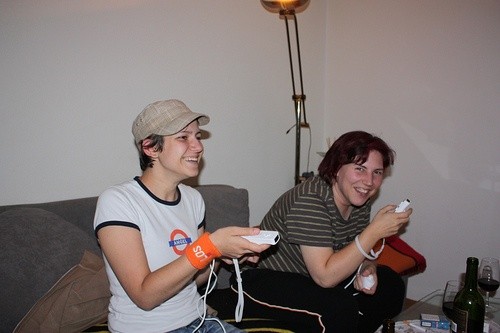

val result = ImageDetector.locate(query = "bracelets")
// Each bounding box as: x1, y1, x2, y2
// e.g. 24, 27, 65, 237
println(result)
185, 232, 223, 270
355, 234, 379, 261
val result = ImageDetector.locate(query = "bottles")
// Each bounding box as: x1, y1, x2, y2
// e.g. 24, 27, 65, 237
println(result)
454, 256, 485, 332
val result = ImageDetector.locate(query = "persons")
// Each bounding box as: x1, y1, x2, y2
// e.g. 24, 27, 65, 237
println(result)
93, 99, 272, 333
229, 131, 413, 333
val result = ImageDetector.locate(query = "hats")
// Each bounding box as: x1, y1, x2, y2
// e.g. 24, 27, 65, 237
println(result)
132, 98, 209, 152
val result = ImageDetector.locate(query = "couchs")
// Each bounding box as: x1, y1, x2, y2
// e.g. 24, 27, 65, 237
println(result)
1, 183, 250, 332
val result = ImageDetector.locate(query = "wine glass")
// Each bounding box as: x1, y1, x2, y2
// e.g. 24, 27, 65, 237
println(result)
443, 279, 466, 322
479, 255, 500, 319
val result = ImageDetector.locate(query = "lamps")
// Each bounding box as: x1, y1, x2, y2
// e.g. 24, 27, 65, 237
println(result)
260, 0, 312, 186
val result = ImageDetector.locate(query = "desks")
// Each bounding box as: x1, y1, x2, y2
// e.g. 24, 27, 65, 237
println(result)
375, 289, 499, 333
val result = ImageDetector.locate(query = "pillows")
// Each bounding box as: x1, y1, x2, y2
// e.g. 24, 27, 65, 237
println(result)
0, 207, 97, 332
